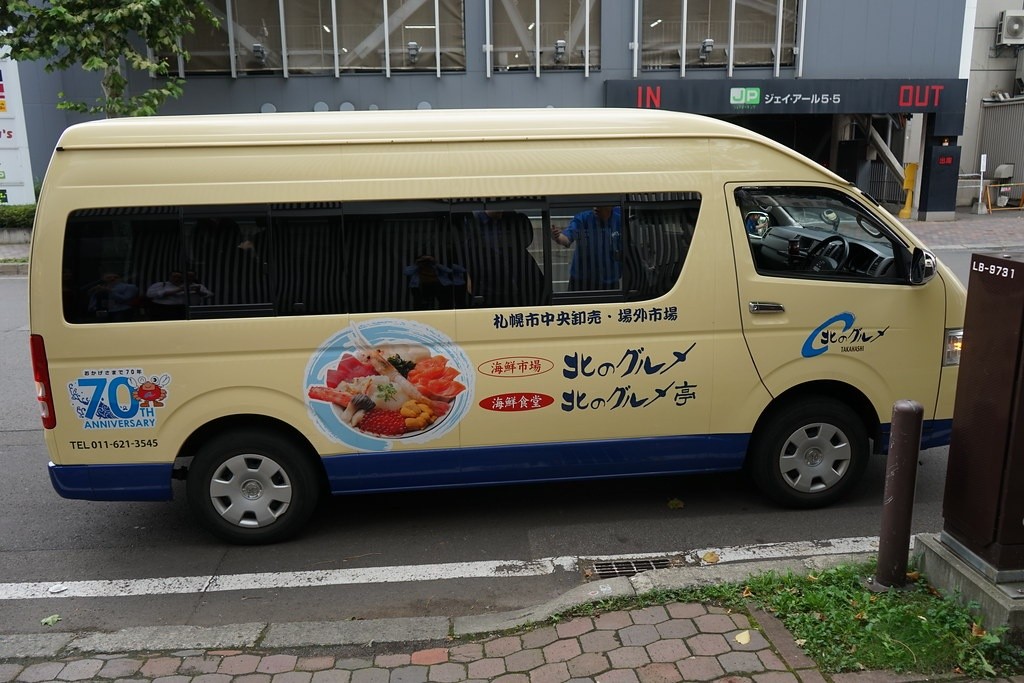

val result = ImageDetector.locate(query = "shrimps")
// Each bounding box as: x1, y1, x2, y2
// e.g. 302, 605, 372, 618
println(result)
346, 320, 451, 418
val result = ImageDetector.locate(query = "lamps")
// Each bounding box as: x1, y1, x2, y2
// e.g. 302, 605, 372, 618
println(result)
699, 38, 714, 61
555, 39, 566, 62
253, 43, 268, 66
407, 40, 419, 64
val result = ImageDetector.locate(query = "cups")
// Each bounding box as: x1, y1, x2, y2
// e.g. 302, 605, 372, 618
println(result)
788, 238, 800, 255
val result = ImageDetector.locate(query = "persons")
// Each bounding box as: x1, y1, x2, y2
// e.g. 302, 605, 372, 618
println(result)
63, 238, 500, 319
551, 201, 624, 290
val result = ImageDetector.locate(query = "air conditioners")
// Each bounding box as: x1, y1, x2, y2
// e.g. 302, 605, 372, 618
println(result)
995, 10, 1024, 47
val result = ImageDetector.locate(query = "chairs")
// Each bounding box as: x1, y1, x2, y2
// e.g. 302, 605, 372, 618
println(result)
512, 214, 546, 297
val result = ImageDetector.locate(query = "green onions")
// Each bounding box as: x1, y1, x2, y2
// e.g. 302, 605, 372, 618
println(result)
375, 384, 398, 402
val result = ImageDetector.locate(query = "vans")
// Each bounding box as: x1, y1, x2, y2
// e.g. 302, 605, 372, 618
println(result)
24, 111, 969, 547
